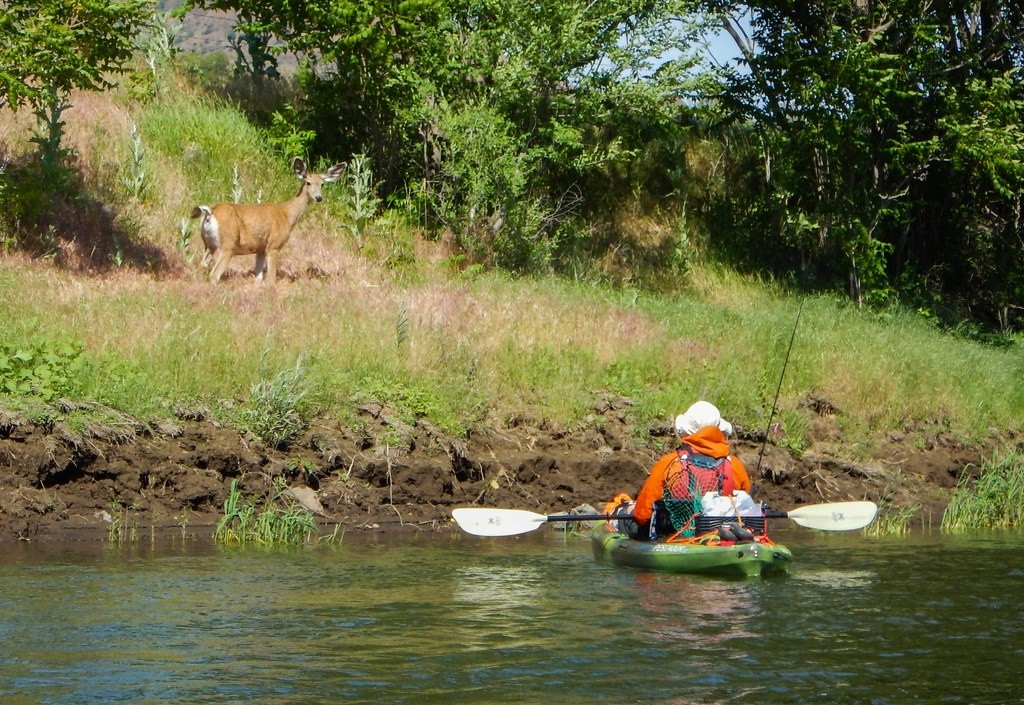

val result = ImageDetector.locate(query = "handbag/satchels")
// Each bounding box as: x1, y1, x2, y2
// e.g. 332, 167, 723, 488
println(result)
701, 489, 760, 516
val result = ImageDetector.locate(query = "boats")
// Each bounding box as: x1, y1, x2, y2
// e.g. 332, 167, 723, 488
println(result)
591, 518, 793, 580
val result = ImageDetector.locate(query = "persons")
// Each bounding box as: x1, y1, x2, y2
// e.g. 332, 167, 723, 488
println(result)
603, 401, 751, 544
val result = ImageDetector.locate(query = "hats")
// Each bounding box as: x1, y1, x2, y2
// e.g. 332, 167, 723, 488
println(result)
676, 400, 732, 438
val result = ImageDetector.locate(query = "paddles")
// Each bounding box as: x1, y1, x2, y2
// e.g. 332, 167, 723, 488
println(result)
452, 500, 877, 538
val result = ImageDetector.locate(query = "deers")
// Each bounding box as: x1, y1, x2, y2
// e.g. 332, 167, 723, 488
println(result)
189, 157, 348, 287
